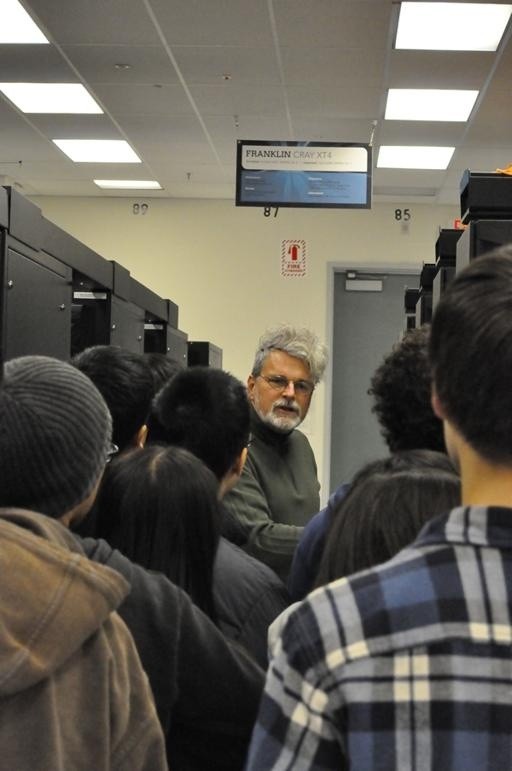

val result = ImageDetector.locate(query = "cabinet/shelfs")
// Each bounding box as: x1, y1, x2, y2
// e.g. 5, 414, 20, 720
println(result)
1, 183, 221, 374
402, 170, 512, 336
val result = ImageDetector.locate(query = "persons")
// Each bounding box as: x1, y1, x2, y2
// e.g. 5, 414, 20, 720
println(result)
1, 246, 512, 771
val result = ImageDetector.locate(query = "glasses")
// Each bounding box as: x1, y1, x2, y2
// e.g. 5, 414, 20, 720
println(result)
107, 442, 119, 463
258, 374, 314, 397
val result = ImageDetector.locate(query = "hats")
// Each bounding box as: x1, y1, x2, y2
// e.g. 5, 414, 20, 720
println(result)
1, 355, 113, 519
